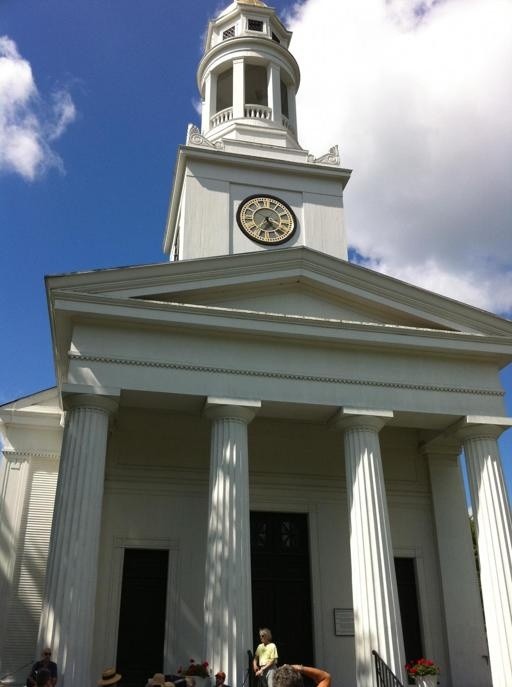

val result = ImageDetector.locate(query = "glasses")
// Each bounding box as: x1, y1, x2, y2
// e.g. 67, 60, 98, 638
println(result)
259, 635, 264, 638
44, 653, 51, 656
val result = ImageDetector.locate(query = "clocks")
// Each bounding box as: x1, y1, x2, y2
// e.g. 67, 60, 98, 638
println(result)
235, 194, 297, 246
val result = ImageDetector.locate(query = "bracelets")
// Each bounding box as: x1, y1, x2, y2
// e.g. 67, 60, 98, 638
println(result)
300, 665, 304, 674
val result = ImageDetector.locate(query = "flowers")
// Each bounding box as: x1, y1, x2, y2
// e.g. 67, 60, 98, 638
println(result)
404, 656, 441, 679
176, 658, 211, 679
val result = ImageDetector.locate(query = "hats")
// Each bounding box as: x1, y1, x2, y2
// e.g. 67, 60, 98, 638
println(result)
215, 672, 225, 679
148, 673, 174, 687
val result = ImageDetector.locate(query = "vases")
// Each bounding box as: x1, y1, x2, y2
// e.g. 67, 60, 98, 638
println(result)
414, 673, 439, 687
185, 674, 211, 687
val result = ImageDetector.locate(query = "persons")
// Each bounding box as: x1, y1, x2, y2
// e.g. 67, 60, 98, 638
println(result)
252, 628, 279, 687
144, 672, 175, 687
39, 670, 53, 687
215, 672, 232, 687
27, 647, 58, 687
185, 660, 199, 676
266, 664, 333, 687
